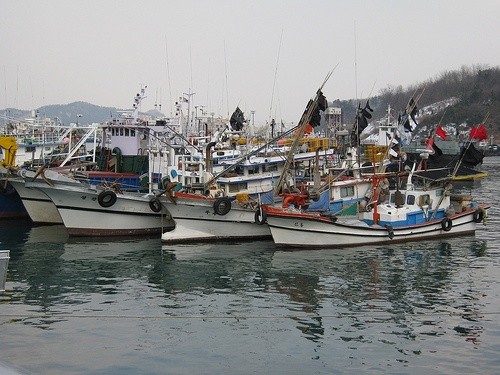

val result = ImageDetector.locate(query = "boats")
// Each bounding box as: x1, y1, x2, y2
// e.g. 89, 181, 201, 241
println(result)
0, 85, 489, 244
260, 63, 492, 248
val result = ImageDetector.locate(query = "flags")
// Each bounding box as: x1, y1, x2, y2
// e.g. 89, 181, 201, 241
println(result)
470, 123, 489, 141
436, 126, 448, 140
